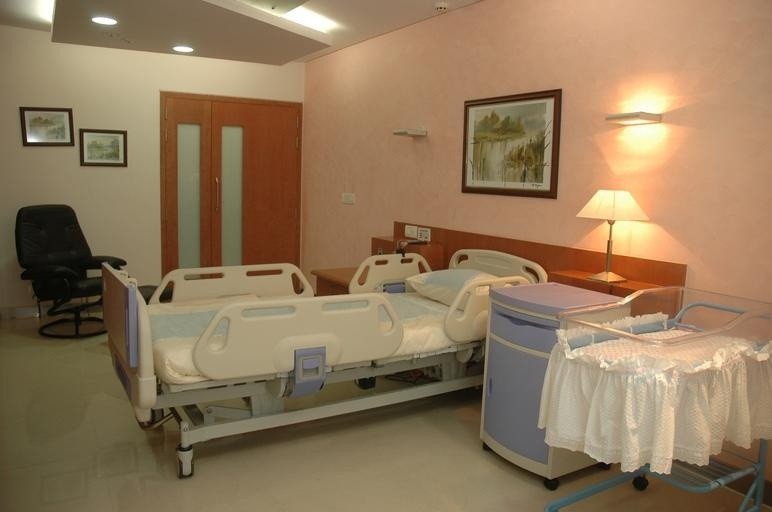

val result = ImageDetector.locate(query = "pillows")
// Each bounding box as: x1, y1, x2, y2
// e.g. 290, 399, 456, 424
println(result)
404, 267, 514, 311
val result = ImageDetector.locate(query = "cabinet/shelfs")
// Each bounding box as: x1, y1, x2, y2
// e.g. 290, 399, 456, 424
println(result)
310, 266, 369, 296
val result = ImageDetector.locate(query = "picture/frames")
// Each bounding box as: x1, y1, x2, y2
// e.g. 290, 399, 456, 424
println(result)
460, 88, 562, 200
19, 106, 74, 147
78, 127, 129, 168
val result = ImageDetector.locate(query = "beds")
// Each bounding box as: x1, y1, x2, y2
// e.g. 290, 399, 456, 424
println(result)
101, 244, 548, 480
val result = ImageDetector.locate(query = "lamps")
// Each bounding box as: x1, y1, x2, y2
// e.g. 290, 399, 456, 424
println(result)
393, 128, 427, 137
604, 110, 661, 127
576, 189, 651, 283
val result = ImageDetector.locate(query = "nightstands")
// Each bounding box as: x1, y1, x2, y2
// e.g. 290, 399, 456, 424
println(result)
478, 282, 631, 493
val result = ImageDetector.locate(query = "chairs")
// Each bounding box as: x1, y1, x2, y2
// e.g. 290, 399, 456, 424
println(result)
15, 204, 127, 339
